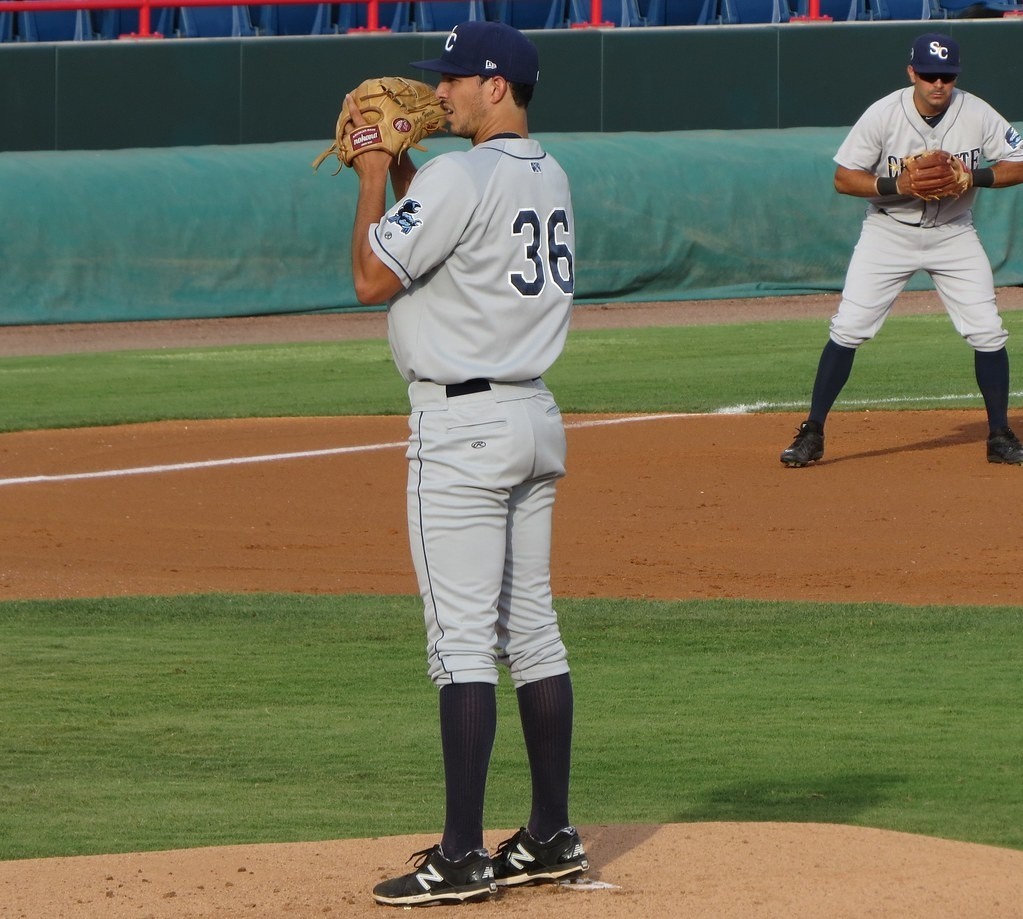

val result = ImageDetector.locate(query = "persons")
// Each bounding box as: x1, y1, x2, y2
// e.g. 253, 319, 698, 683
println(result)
780, 32, 1023, 468
343, 23, 590, 905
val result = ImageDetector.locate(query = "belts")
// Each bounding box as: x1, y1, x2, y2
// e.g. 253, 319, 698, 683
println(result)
446, 379, 492, 398
879, 209, 922, 228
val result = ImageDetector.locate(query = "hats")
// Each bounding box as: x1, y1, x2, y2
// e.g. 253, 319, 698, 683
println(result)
910, 32, 960, 73
411, 19, 539, 88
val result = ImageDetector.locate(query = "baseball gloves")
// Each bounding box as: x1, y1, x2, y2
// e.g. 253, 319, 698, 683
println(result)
312, 76, 448, 176
901, 149, 974, 201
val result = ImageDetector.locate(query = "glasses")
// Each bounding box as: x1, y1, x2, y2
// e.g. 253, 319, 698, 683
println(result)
917, 73, 958, 83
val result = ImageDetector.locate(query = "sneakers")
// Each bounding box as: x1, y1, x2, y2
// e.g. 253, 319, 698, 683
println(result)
373, 843, 496, 906
488, 827, 590, 886
780, 421, 825, 468
987, 428, 1023, 464
